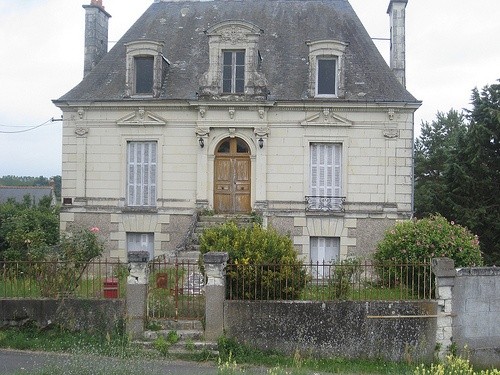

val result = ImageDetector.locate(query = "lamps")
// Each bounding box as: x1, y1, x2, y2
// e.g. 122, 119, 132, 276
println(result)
258, 138, 264, 149
199, 137, 204, 148
